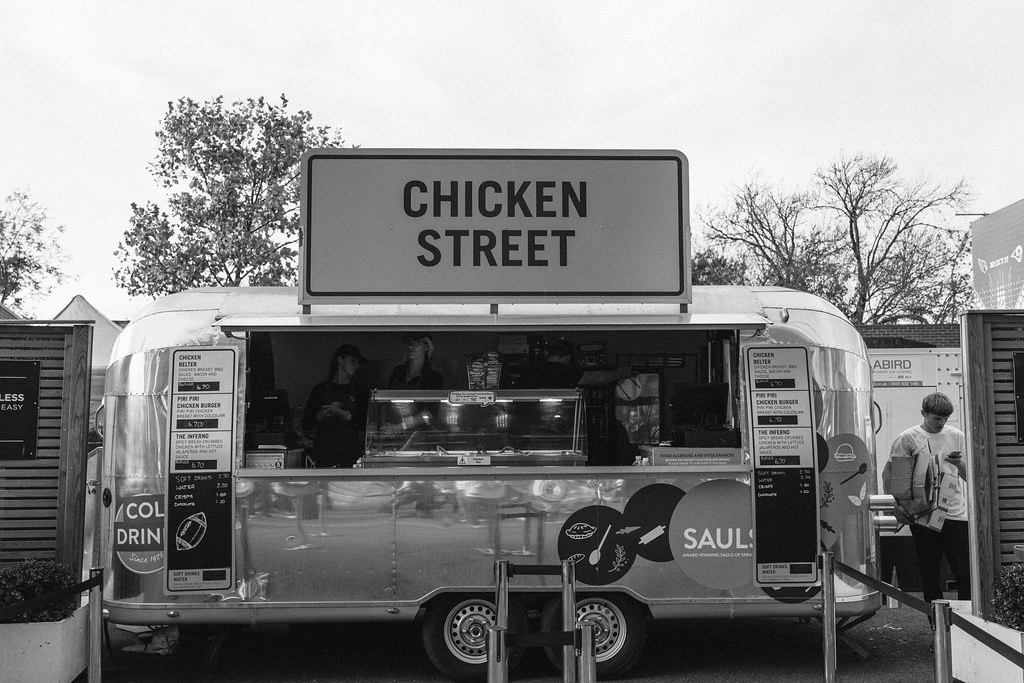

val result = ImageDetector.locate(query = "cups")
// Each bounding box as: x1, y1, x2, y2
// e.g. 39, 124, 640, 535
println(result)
466, 361, 486, 390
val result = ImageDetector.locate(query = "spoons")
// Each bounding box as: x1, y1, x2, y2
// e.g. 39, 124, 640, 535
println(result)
589, 525, 612, 565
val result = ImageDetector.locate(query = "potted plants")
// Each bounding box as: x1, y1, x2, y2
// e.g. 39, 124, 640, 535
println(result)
0, 557, 90, 683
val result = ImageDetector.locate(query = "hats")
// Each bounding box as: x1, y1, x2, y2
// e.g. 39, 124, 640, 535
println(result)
400, 332, 432, 342
335, 344, 366, 360
555, 339, 575, 357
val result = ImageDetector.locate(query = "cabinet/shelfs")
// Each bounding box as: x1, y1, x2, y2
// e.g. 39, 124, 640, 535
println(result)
245, 449, 304, 468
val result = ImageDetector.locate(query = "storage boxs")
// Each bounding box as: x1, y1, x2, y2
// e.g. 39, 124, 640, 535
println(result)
638, 445, 741, 466
467, 362, 503, 390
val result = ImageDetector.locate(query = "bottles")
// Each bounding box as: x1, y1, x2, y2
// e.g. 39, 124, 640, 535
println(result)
598, 414, 605, 435
486, 352, 502, 389
591, 410, 598, 436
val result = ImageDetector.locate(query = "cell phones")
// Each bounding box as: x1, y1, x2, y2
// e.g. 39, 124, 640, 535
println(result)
949, 451, 961, 458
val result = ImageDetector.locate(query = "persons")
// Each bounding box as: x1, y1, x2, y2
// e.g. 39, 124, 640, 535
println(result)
302, 344, 371, 468
883, 393, 971, 653
509, 341, 639, 457
335, 332, 445, 457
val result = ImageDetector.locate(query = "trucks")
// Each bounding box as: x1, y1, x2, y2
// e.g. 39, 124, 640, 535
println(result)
94, 284, 899, 683
